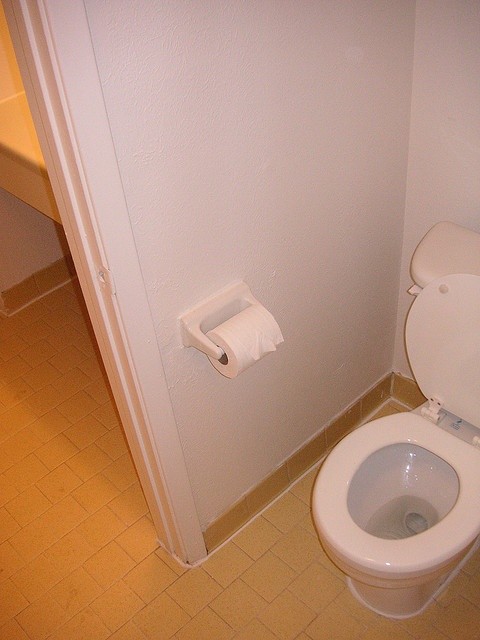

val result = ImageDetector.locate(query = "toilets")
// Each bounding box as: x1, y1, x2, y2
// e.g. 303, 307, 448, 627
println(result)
310, 221, 480, 619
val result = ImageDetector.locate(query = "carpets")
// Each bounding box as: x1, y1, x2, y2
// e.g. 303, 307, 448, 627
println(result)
0, 185, 77, 317
